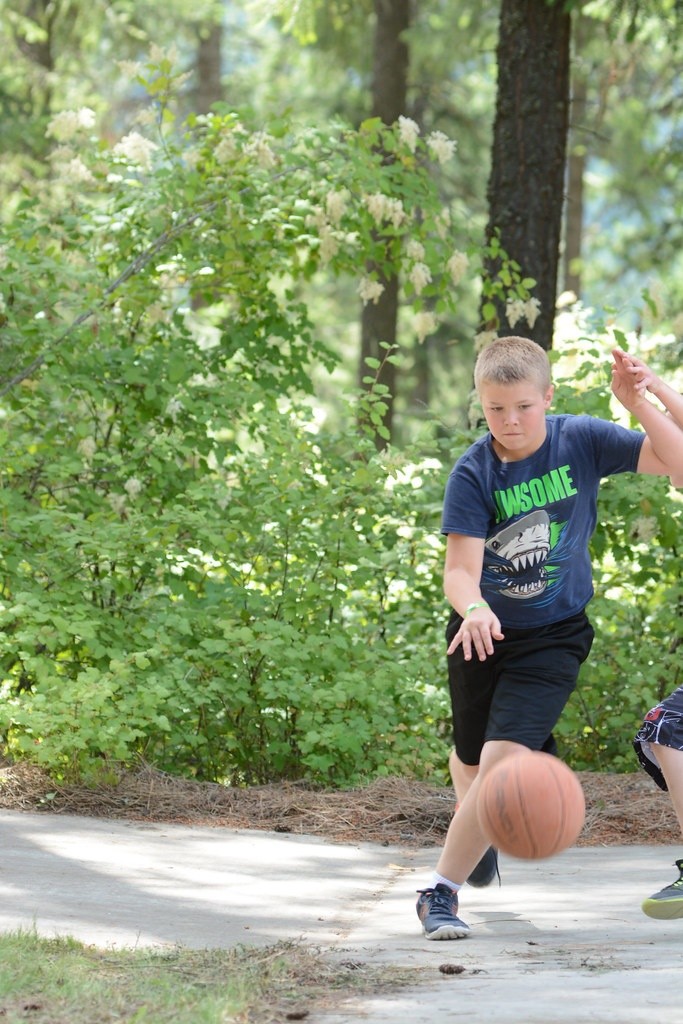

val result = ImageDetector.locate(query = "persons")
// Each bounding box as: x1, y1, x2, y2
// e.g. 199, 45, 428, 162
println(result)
615, 349, 683, 919
416, 337, 682, 939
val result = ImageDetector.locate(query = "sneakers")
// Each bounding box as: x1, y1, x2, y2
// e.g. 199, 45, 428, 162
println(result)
454, 801, 502, 889
416, 882, 470, 940
641, 859, 683, 920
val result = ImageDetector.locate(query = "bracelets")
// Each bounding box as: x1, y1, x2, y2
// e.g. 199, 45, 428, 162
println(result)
464, 603, 488, 618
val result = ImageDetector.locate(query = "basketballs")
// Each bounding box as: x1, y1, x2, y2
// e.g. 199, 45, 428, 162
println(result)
478, 748, 590, 860
